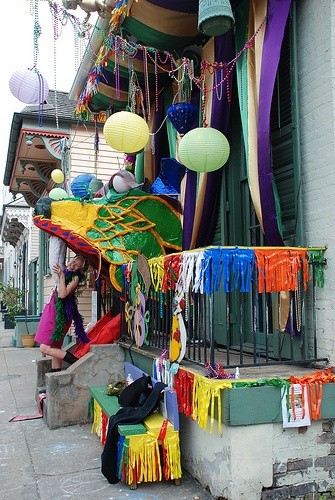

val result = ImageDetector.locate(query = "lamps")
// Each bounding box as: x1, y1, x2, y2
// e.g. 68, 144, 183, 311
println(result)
197, 0, 235, 36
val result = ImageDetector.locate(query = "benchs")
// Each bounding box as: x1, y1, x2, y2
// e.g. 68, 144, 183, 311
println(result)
88, 361, 183, 490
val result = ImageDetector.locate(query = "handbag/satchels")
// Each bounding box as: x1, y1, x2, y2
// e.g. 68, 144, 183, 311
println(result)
118, 373, 154, 408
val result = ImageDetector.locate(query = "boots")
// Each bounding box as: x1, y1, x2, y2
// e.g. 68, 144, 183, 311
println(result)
39, 368, 61, 393
62, 352, 78, 365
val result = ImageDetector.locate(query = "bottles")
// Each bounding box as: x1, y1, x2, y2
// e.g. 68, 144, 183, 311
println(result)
126, 374, 131, 387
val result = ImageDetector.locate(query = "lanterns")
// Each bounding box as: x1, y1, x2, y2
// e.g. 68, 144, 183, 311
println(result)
113, 171, 134, 193
9, 68, 49, 104
103, 111, 150, 154
70, 174, 96, 198
51, 169, 65, 184
49, 188, 68, 200
178, 127, 230, 174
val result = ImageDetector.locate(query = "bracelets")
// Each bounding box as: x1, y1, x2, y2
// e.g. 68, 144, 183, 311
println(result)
60, 276, 65, 278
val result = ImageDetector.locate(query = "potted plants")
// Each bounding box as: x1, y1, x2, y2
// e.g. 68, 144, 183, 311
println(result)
0, 275, 36, 347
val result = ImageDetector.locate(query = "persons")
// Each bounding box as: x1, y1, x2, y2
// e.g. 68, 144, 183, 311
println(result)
62, 0, 114, 24
36, 255, 89, 373
